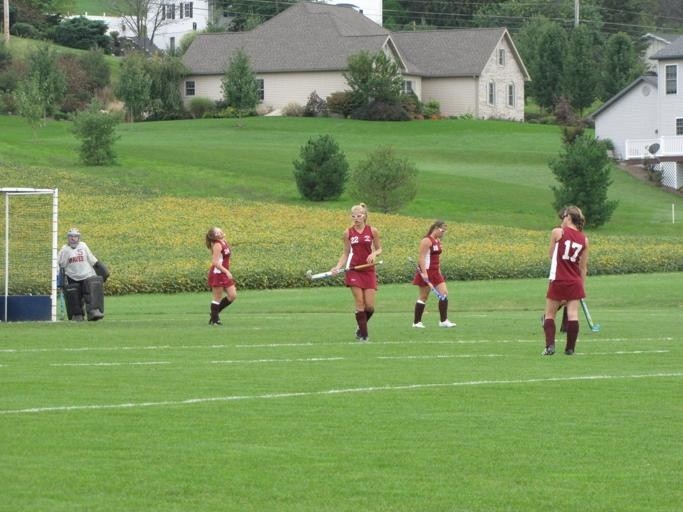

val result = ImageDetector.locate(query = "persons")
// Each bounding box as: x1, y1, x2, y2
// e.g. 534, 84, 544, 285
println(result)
412, 219, 457, 329
540, 204, 588, 357
331, 203, 383, 343
55, 227, 111, 322
205, 226, 238, 326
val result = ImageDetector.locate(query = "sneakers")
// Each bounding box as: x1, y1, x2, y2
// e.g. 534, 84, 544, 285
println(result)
412, 322, 424, 328
541, 345, 554, 355
209, 318, 221, 326
354, 328, 367, 341
439, 319, 455, 327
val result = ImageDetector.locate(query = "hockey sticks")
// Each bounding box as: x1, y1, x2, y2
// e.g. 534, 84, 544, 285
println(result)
408, 256, 448, 302
581, 298, 600, 332
307, 261, 384, 279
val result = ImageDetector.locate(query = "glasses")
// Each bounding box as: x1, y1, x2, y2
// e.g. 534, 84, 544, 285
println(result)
351, 213, 365, 219
